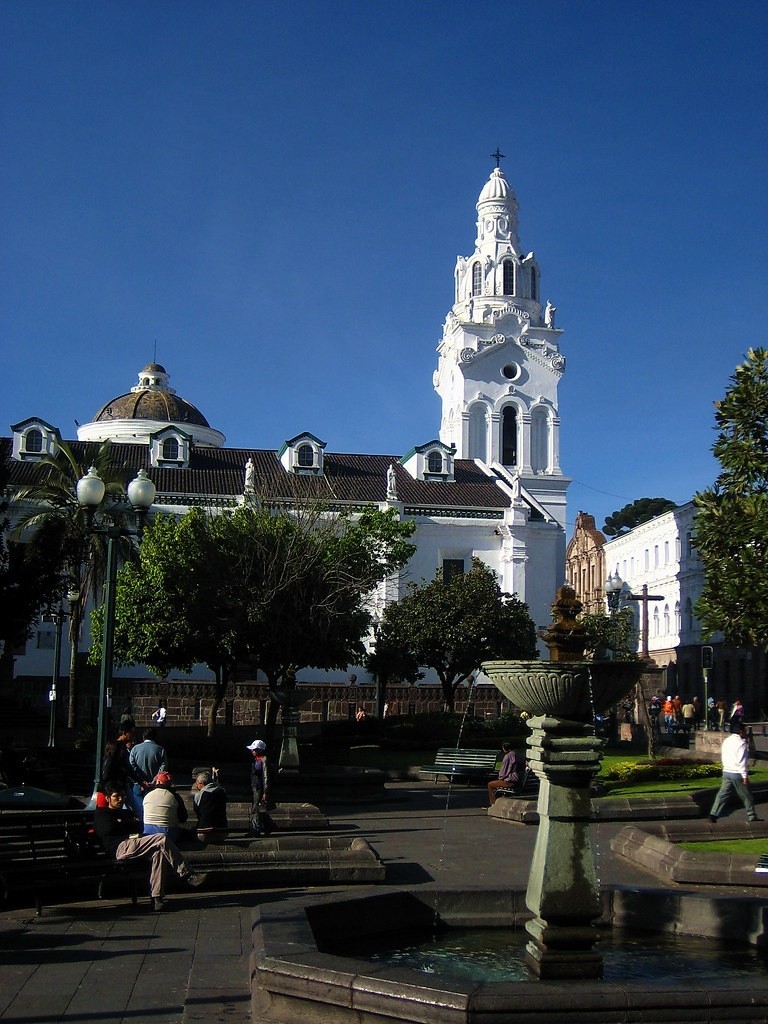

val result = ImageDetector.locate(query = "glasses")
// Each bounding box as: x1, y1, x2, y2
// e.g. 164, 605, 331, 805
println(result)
116, 794, 127, 799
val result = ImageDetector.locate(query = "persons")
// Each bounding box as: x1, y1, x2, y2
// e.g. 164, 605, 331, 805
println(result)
97, 702, 209, 911
242, 740, 276, 838
356, 706, 366, 722
383, 699, 401, 719
709, 721, 764, 823
481, 742, 525, 810
648, 694, 743, 733
190, 771, 228, 843
545, 300, 557, 328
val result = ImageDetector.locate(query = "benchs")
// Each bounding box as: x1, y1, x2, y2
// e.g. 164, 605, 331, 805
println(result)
492, 769, 540, 799
0, 810, 152, 917
419, 748, 501, 790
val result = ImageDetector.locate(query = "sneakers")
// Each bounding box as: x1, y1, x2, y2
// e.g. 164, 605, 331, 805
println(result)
150, 896, 168, 911
183, 873, 208, 888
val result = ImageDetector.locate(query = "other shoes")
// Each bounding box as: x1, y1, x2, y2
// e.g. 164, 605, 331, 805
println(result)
708, 814, 719, 823
245, 832, 261, 837
751, 818, 765, 822
264, 824, 279, 838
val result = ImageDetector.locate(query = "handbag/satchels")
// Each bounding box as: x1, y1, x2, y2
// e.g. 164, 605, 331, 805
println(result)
259, 798, 277, 812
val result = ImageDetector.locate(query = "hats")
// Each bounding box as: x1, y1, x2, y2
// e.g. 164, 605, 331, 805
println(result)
246, 739, 266, 751
667, 696, 671, 702
